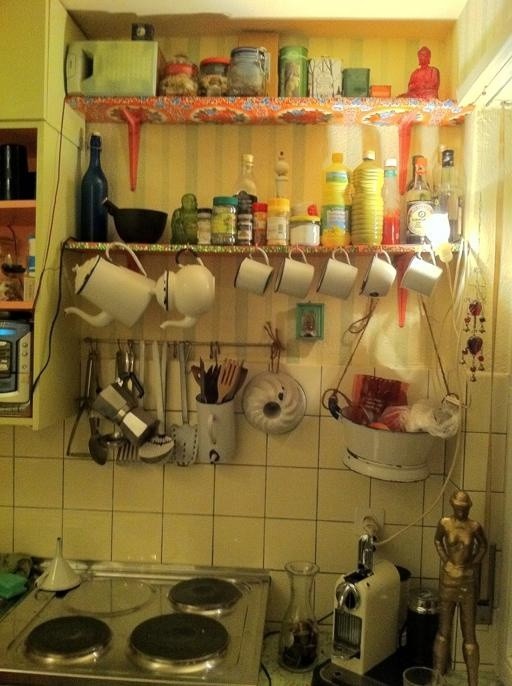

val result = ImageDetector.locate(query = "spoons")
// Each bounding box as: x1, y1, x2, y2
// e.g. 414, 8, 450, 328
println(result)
89, 352, 110, 465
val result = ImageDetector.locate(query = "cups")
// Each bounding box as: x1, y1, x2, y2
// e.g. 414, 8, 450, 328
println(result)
195, 394, 237, 465
234, 245, 443, 297
92, 373, 160, 447
403, 666, 442, 686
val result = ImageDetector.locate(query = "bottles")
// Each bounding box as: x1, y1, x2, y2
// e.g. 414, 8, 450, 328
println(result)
80, 134, 105, 244
275, 151, 289, 200
233, 156, 259, 243
292, 200, 320, 245
156, 44, 309, 95
321, 144, 459, 244
405, 588, 447, 676
171, 196, 265, 244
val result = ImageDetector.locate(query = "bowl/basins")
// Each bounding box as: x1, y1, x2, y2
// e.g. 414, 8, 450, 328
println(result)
104, 199, 168, 242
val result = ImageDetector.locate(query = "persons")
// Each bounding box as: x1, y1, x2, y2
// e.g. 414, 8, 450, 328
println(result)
396, 47, 440, 100
432, 490, 488, 686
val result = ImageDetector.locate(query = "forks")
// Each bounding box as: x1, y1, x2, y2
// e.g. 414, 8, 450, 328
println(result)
207, 357, 246, 404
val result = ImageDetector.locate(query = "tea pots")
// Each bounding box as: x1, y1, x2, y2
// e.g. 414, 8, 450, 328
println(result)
62, 243, 217, 330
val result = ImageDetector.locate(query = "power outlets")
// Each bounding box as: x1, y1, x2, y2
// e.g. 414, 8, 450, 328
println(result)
354, 507, 384, 550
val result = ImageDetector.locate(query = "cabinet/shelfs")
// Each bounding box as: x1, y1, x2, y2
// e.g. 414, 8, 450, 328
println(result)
62, 94, 463, 254
0, 0, 82, 430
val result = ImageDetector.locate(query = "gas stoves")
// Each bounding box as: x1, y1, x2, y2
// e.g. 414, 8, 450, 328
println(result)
1, 574, 271, 686
